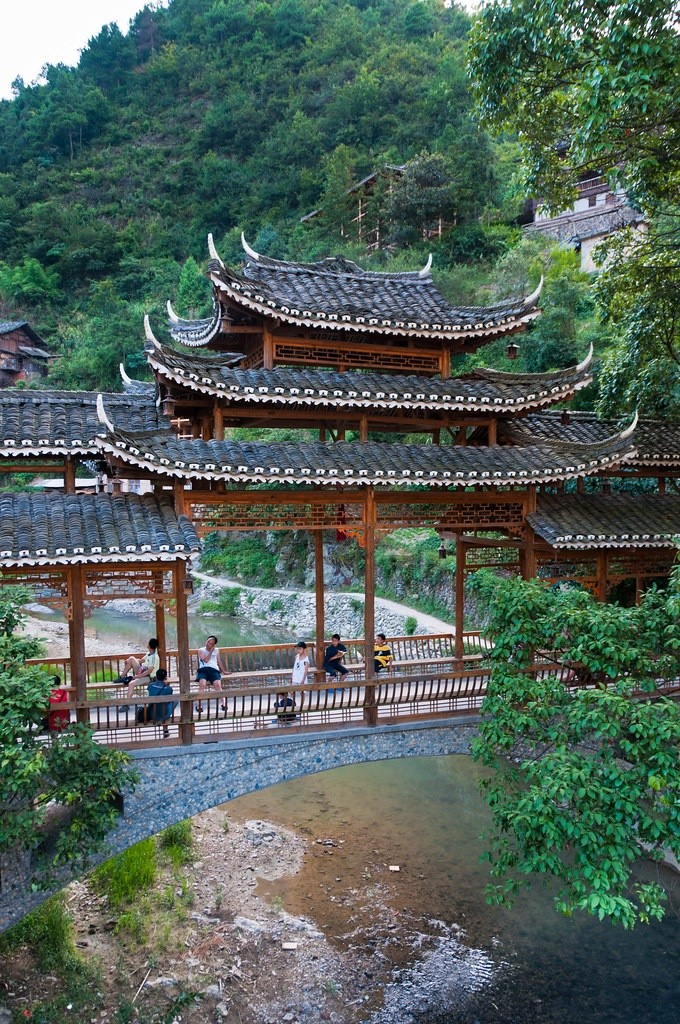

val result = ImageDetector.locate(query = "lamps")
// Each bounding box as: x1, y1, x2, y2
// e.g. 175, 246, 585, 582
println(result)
559, 400, 573, 426
182, 560, 195, 600
159, 386, 178, 415
438, 541, 455, 560
506, 333, 520, 359
547, 553, 564, 579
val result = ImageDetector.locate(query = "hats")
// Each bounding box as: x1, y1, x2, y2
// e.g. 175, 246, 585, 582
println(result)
293, 642, 306, 650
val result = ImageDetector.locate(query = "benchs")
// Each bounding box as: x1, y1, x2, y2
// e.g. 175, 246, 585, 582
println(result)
16, 662, 607, 755
24, 630, 575, 682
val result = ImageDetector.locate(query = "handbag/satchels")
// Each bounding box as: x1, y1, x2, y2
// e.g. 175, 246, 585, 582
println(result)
39, 716, 49, 734
137, 707, 152, 724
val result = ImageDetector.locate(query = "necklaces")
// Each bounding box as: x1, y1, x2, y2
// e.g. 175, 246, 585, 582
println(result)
147, 651, 155, 657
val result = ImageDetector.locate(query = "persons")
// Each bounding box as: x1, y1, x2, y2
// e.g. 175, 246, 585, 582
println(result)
38, 675, 71, 734
323, 634, 349, 693
148, 669, 179, 738
195, 635, 232, 712
290, 642, 310, 707
275, 692, 297, 722
112, 638, 160, 712
362, 634, 393, 678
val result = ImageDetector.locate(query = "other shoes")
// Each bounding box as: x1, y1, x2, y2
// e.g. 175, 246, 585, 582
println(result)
196, 703, 203, 712
221, 705, 228, 711
114, 677, 125, 683
119, 705, 130, 713
328, 689, 334, 693
164, 731, 170, 738
336, 688, 342, 691
